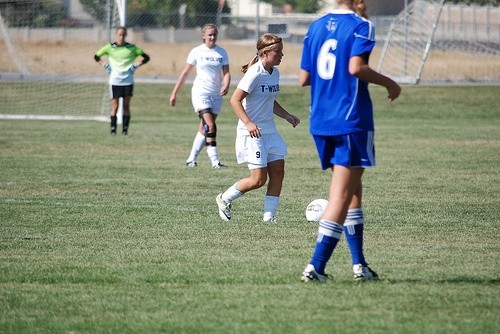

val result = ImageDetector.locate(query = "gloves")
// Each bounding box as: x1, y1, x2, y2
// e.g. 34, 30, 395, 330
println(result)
128, 65, 135, 73
104, 64, 111, 71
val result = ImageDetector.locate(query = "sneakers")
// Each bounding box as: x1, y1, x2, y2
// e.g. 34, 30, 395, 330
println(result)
301, 264, 335, 285
215, 193, 232, 222
352, 263, 379, 281
264, 211, 278, 223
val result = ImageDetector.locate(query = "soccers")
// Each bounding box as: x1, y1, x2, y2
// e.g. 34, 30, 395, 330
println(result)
305, 198, 328, 222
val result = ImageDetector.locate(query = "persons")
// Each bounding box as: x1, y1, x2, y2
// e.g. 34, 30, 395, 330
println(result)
356, 0, 367, 19
299, 0, 402, 282
94, 27, 150, 135
170, 24, 231, 169
216, 34, 300, 222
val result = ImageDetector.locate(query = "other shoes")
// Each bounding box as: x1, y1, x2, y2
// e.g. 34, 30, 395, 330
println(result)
213, 161, 228, 169
185, 160, 198, 167
